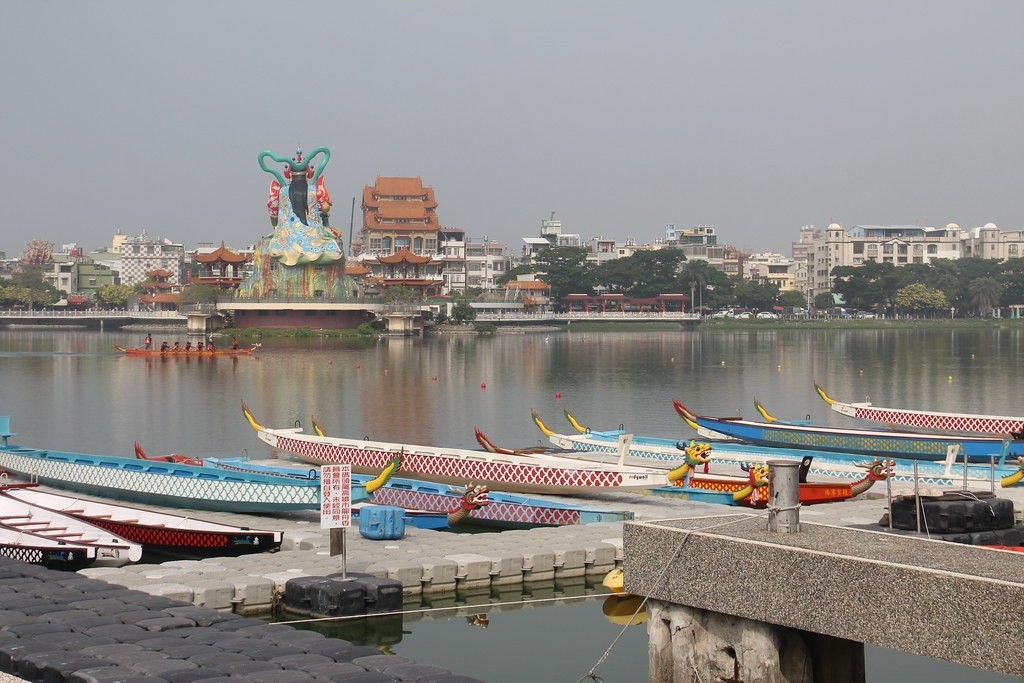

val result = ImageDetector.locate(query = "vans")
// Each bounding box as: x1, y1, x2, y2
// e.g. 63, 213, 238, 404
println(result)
715, 311, 734, 318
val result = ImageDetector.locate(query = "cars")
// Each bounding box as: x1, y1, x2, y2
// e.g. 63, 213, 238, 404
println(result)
734, 312, 753, 318
858, 311, 875, 319
756, 311, 776, 319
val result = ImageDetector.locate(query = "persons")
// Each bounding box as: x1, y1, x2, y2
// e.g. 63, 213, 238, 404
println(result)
196, 342, 203, 352
145, 334, 151, 349
208, 342, 212, 351
172, 342, 182, 352
184, 342, 195, 352
160, 342, 170, 352
231, 337, 238, 350
236, 145, 362, 268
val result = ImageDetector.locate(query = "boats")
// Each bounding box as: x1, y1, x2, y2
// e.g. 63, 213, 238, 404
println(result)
813, 383, 1024, 438
116, 343, 263, 356
1, 397, 1024, 570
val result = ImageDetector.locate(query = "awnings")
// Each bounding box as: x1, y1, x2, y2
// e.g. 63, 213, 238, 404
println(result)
67, 295, 86, 304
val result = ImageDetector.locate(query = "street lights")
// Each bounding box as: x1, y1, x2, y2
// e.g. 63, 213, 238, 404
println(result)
691, 281, 698, 317
